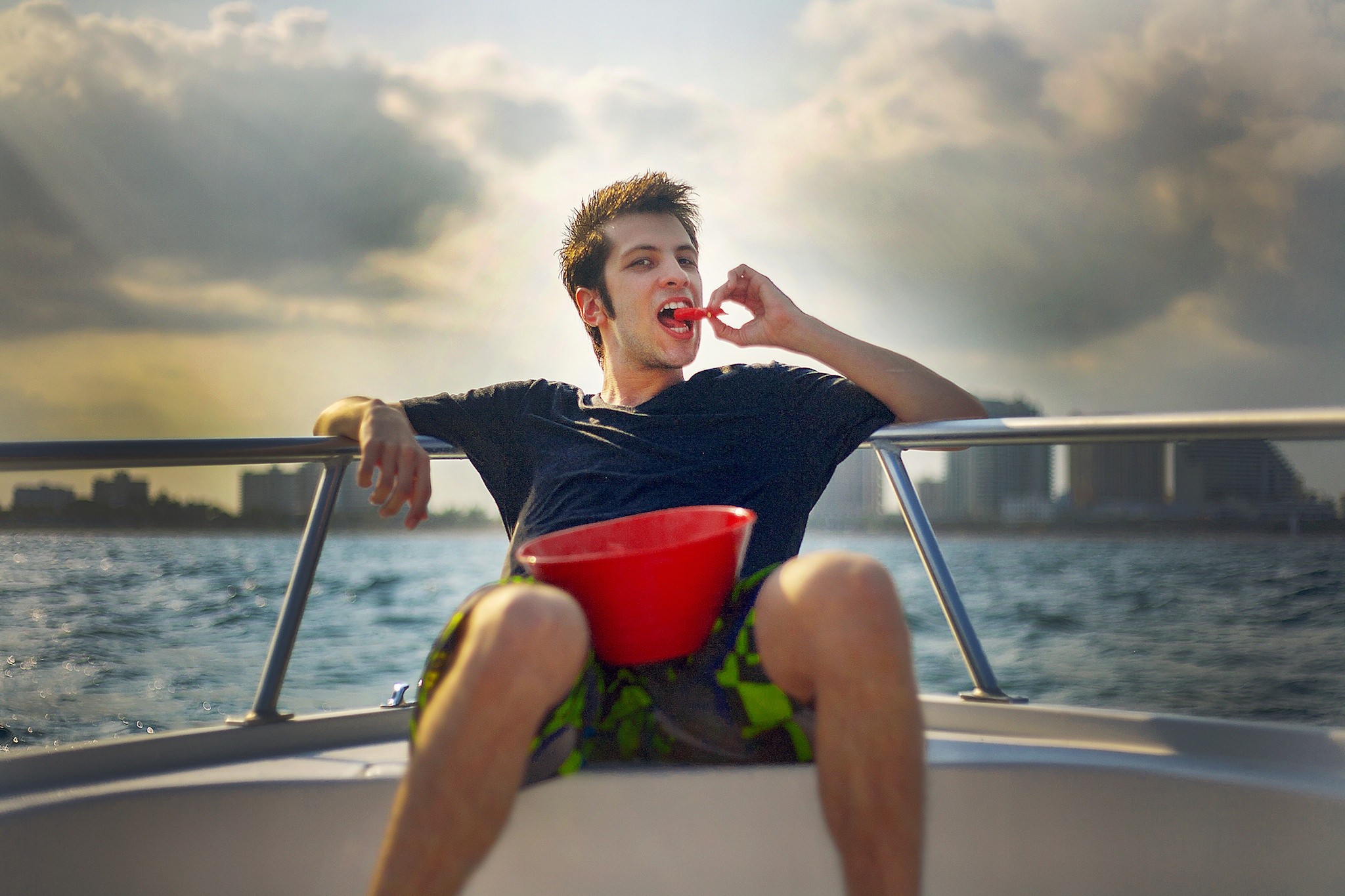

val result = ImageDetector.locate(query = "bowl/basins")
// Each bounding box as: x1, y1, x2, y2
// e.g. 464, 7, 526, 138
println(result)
516, 505, 757, 665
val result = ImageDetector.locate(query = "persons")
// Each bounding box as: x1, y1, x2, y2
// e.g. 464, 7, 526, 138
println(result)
312, 169, 991, 896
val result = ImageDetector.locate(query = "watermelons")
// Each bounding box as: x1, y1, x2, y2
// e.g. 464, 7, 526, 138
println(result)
673, 308, 725, 321
